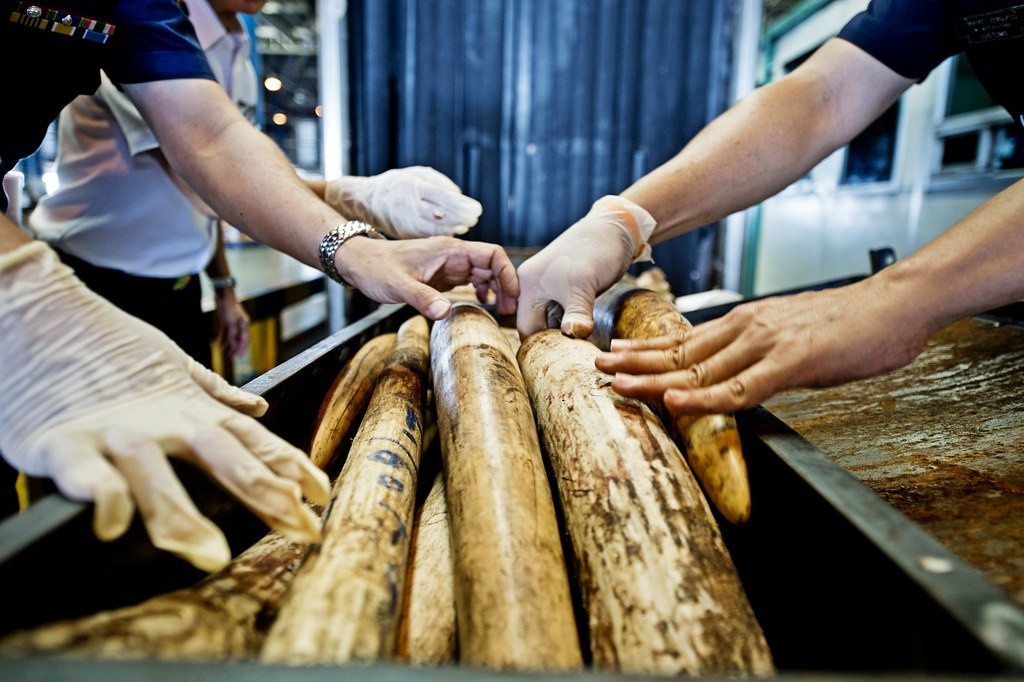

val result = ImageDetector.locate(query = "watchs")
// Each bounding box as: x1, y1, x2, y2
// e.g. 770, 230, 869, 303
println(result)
318, 220, 387, 287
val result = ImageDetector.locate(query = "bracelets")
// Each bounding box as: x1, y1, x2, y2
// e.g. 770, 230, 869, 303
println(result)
214, 278, 235, 287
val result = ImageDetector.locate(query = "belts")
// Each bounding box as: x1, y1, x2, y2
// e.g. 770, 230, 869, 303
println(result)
68, 256, 195, 292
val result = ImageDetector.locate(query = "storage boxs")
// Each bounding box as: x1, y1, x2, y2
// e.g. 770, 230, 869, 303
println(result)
0, 245, 1024, 682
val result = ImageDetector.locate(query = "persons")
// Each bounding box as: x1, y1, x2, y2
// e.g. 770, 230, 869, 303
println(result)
26, 0, 483, 513
517, 0, 1024, 411
0, 0, 518, 570
